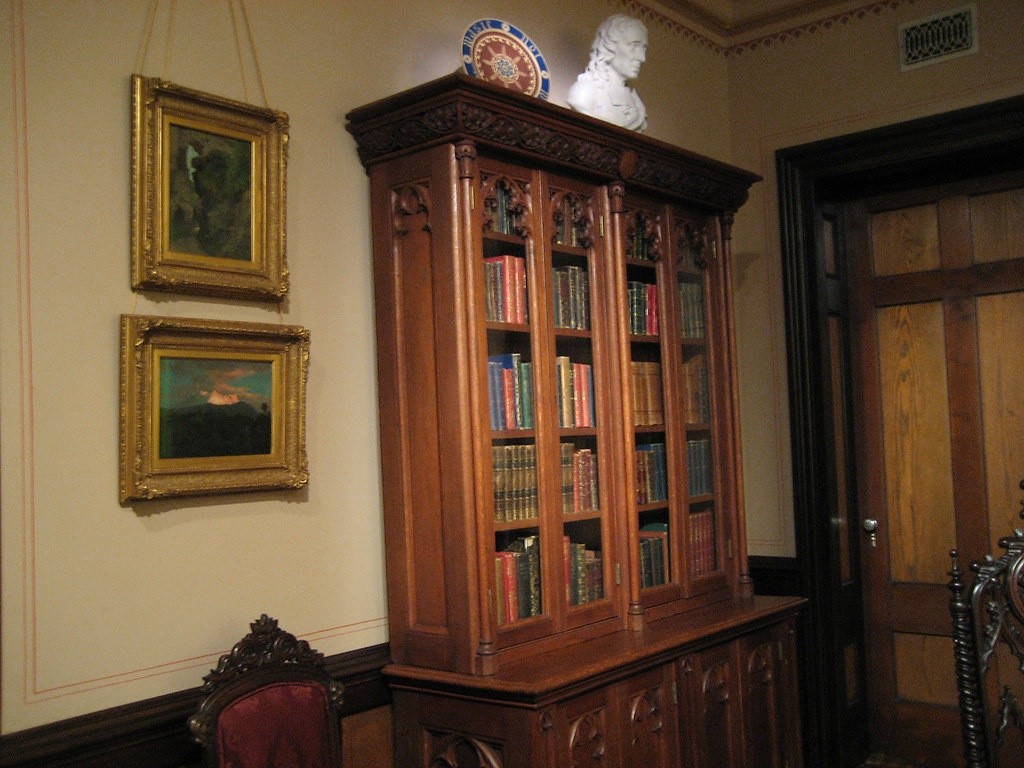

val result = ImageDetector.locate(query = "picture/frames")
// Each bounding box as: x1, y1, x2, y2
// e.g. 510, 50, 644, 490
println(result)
130, 73, 293, 301
118, 313, 309, 503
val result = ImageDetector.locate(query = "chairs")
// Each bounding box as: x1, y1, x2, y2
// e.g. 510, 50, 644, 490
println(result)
185, 614, 348, 768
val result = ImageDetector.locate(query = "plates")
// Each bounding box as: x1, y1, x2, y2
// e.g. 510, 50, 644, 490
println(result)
461, 18, 550, 101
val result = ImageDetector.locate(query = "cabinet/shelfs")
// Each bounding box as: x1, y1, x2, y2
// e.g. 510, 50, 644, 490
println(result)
342, 72, 810, 768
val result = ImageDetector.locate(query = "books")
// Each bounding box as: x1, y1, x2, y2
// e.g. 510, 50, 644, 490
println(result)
478, 255, 718, 628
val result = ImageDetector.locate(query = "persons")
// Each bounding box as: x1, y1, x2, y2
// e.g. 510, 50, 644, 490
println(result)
565, 14, 649, 136
